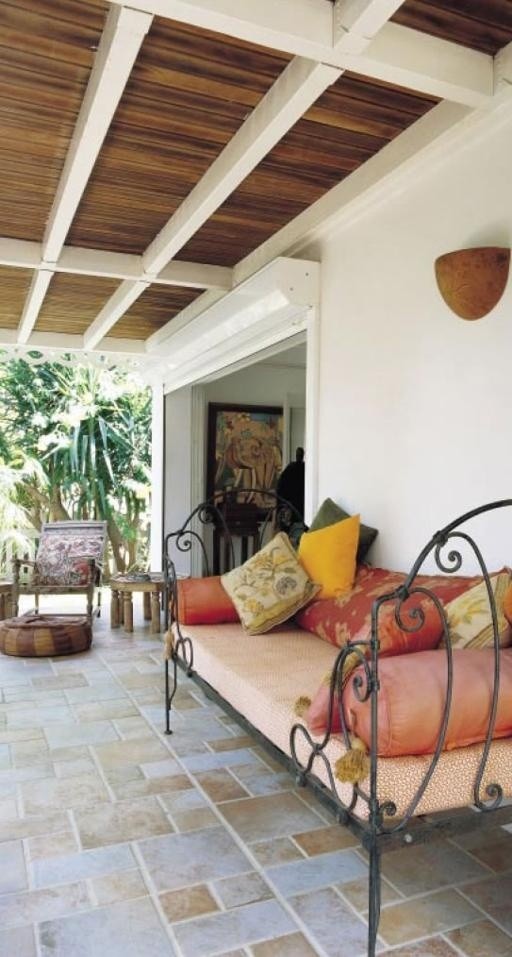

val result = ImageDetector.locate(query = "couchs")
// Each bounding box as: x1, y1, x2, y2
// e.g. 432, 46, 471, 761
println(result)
160, 485, 512, 955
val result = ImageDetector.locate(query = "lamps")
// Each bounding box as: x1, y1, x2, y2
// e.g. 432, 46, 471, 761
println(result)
429, 242, 511, 321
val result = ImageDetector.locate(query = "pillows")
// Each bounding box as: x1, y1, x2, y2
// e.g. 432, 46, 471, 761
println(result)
437, 571, 511, 650
304, 497, 378, 562
293, 564, 487, 656
338, 655, 512, 751
298, 512, 363, 598
220, 532, 318, 631
309, 600, 443, 741
177, 574, 244, 625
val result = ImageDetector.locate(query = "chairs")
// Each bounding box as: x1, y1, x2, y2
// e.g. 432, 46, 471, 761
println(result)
11, 519, 109, 622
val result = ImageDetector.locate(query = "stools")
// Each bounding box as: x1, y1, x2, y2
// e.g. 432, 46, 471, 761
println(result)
109, 573, 168, 634
